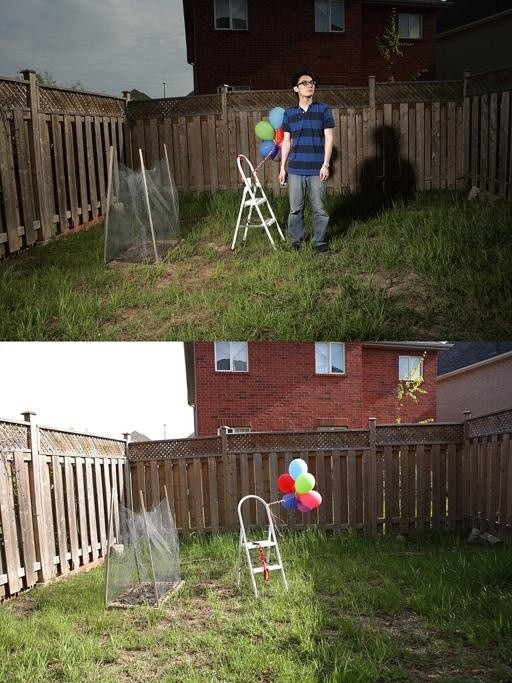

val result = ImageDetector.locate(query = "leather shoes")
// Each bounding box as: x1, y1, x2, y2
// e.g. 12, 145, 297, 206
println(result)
312, 244, 331, 253
290, 244, 299, 253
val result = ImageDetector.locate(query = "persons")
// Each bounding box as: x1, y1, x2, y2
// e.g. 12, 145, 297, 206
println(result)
278, 70, 336, 254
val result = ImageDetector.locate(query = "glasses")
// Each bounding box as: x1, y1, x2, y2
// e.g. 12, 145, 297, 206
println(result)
297, 80, 316, 87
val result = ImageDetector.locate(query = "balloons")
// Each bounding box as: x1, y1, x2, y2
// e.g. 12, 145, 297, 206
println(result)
274, 455, 323, 515
254, 105, 294, 161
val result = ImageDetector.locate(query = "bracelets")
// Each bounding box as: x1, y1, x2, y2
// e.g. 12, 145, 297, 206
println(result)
322, 163, 330, 169
